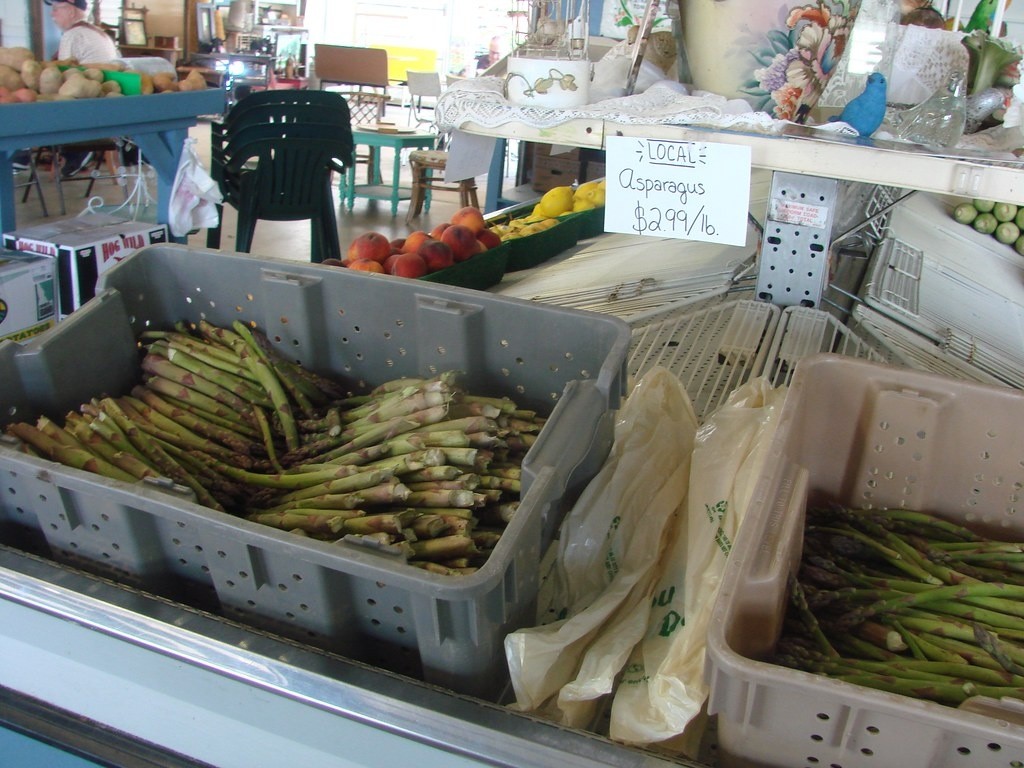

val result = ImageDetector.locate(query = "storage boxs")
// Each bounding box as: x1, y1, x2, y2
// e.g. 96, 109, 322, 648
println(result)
1, 243, 634, 704
707, 349, 1023, 768
1, 243, 58, 352
2, 210, 170, 323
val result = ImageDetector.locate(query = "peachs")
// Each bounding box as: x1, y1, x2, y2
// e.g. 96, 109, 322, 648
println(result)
320, 206, 500, 278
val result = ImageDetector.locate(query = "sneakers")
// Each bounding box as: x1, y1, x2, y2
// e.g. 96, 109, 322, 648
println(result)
61, 151, 94, 176
11, 156, 31, 171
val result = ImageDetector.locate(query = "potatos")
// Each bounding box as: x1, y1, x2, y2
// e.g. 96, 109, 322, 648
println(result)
0, 46, 209, 105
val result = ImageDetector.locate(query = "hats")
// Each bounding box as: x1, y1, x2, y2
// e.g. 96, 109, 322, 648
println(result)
43, 0, 90, 11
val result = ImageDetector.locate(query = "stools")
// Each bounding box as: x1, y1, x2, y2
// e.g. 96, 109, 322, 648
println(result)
404, 150, 480, 226
54, 138, 132, 216
13, 146, 53, 220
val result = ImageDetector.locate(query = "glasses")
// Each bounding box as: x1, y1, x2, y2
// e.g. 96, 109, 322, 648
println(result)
53, 4, 73, 11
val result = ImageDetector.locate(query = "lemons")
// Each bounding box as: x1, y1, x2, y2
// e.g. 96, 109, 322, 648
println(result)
526, 180, 605, 223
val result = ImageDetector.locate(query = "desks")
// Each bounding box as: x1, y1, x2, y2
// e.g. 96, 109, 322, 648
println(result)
338, 129, 438, 217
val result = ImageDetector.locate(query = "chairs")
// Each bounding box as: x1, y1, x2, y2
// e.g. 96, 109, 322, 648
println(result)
407, 70, 442, 133
205, 89, 355, 266
339, 91, 391, 190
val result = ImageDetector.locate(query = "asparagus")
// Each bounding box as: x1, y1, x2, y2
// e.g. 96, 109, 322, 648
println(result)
760, 488, 1022, 712
2, 319, 547, 586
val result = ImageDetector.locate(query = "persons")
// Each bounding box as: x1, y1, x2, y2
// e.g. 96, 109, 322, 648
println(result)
458, 36, 509, 80
12, 0, 122, 178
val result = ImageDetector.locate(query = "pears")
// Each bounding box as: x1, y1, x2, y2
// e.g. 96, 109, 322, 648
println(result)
487, 213, 560, 241
